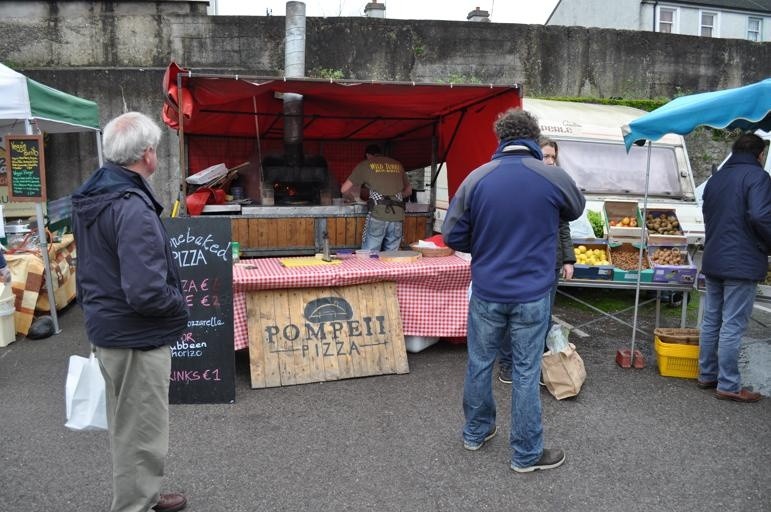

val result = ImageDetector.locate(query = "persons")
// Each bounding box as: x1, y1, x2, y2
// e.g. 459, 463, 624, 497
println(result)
696, 132, 771, 402
340, 146, 415, 251
499, 133, 576, 389
69, 111, 194, 511
441, 112, 586, 473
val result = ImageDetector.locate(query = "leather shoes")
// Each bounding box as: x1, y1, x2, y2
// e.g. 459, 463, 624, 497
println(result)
714, 385, 763, 403
695, 379, 719, 390
151, 493, 188, 512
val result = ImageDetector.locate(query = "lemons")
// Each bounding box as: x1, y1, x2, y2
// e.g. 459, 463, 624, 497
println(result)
574, 245, 609, 266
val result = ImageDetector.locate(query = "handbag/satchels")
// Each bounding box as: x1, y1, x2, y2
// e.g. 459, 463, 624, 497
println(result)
62, 340, 110, 434
540, 342, 587, 401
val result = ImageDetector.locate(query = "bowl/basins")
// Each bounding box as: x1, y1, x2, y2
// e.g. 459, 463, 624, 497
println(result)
338, 250, 353, 260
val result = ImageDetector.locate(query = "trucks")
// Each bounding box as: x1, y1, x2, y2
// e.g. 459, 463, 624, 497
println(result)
420, 96, 705, 305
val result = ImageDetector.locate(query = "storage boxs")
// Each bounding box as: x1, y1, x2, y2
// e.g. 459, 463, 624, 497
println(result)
565, 198, 697, 286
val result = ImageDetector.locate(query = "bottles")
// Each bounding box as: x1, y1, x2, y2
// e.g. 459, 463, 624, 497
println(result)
231, 186, 245, 200
262, 184, 275, 206
232, 241, 241, 262
323, 230, 332, 262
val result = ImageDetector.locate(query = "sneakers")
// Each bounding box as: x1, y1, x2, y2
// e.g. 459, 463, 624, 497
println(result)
463, 424, 498, 451
498, 365, 513, 385
539, 369, 547, 386
509, 446, 567, 474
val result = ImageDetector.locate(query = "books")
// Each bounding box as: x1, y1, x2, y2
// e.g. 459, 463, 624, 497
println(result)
377, 251, 419, 262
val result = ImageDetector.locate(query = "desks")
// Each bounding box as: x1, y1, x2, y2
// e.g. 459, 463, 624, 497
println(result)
549, 278, 692, 347
234, 250, 480, 353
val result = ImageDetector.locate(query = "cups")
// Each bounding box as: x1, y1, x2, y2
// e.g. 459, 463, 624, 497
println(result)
320, 189, 332, 206
333, 199, 345, 205
357, 249, 370, 262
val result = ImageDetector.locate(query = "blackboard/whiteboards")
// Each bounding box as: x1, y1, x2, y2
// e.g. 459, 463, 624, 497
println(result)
5, 135, 46, 202
161, 217, 235, 404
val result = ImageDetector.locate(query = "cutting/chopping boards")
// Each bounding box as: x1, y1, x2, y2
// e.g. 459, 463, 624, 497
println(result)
379, 251, 424, 262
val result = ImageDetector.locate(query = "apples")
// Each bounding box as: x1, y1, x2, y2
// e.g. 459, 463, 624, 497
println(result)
610, 216, 638, 227
